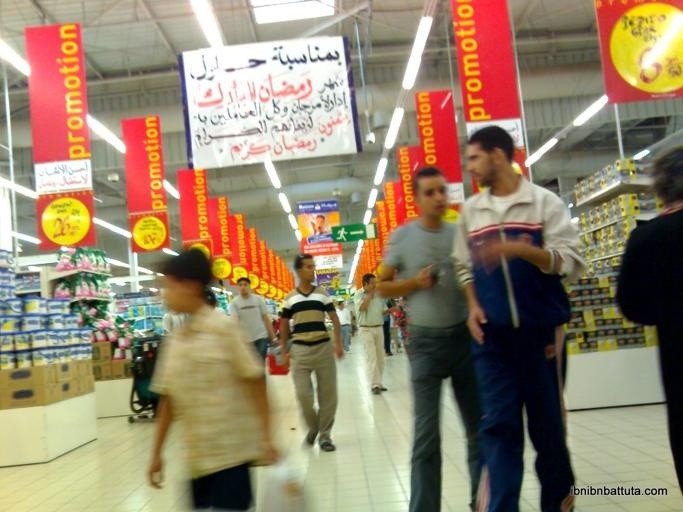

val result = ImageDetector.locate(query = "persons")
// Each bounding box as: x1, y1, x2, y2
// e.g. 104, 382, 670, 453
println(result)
377, 166, 487, 512
231, 278, 280, 379
278, 254, 342, 451
310, 213, 331, 237
335, 273, 396, 395
614, 146, 683, 494
162, 308, 185, 335
450, 126, 587, 512
206, 295, 226, 315
147, 248, 279, 511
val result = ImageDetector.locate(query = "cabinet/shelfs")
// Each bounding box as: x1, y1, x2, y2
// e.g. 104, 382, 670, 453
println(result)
14, 267, 115, 303
575, 177, 659, 264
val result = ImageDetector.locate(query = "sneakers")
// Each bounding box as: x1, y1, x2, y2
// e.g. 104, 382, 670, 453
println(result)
371, 386, 391, 395
304, 431, 318, 446
319, 442, 336, 451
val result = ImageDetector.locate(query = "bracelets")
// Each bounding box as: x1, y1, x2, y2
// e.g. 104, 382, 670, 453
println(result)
272, 337, 277, 342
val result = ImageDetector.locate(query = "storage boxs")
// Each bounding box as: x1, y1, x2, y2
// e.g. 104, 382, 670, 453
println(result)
91, 341, 134, 381
0, 356, 96, 412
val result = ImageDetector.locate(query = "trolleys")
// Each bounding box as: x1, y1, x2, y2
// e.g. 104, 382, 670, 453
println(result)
128, 336, 163, 423
390, 315, 404, 354
